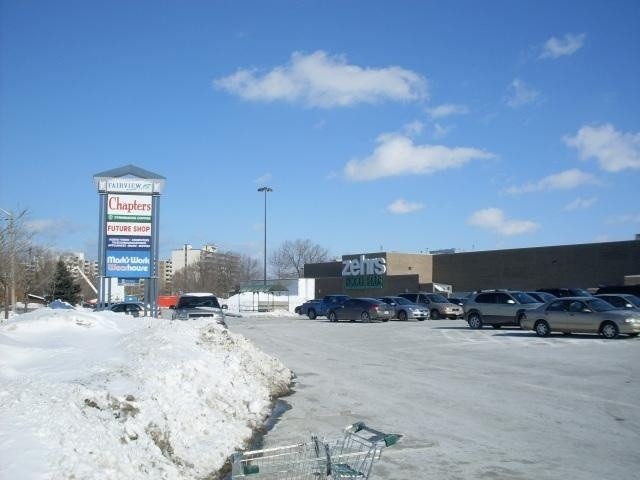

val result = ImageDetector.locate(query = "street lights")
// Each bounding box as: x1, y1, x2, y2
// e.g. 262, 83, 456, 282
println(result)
257, 186, 275, 285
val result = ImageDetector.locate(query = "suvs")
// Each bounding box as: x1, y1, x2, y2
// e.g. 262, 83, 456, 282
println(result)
168, 292, 229, 327
462, 289, 543, 329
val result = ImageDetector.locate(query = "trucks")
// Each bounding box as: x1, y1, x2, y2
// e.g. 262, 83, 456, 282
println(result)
594, 283, 639, 297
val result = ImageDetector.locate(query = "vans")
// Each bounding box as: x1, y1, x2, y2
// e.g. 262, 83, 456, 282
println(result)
538, 287, 593, 299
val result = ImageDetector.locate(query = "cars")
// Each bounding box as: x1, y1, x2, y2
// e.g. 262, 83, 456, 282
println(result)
293, 288, 473, 328
84, 300, 161, 318
525, 291, 559, 304
520, 296, 640, 338
589, 293, 640, 313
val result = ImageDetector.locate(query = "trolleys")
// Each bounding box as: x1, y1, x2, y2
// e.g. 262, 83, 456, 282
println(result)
226, 415, 402, 480
